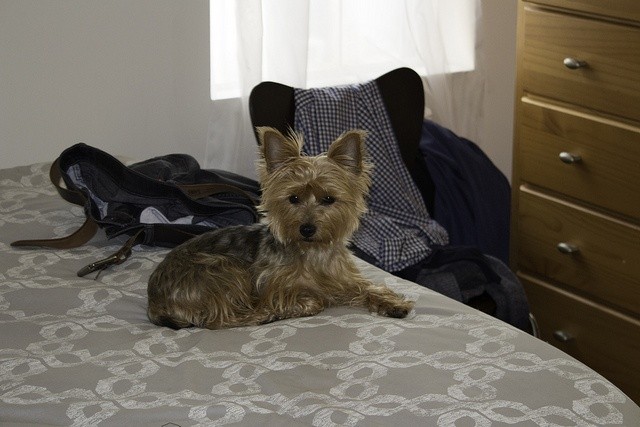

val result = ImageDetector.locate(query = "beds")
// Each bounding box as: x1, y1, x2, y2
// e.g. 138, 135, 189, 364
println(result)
0, 152, 640, 425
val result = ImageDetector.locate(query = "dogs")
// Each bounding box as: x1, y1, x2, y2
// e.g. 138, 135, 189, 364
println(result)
145, 124, 417, 332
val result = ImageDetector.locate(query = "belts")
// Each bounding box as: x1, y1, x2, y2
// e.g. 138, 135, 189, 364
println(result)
10, 142, 263, 280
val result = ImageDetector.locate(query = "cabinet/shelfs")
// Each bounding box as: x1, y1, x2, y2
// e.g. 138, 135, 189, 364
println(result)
508, 1, 640, 404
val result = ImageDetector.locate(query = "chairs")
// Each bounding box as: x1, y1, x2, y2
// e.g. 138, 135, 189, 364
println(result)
248, 66, 541, 340
